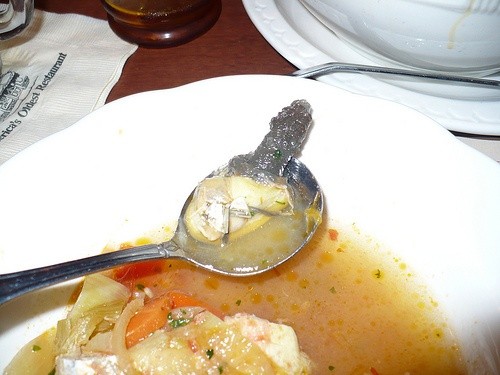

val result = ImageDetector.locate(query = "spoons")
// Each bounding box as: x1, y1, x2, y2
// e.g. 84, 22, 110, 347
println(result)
0, 152, 323, 303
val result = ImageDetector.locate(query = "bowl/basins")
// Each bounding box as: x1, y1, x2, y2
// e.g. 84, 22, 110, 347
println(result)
302, 1, 500, 78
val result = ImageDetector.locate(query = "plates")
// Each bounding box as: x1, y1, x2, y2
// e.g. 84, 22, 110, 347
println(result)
241, 0, 500, 137
0, 73, 500, 375
